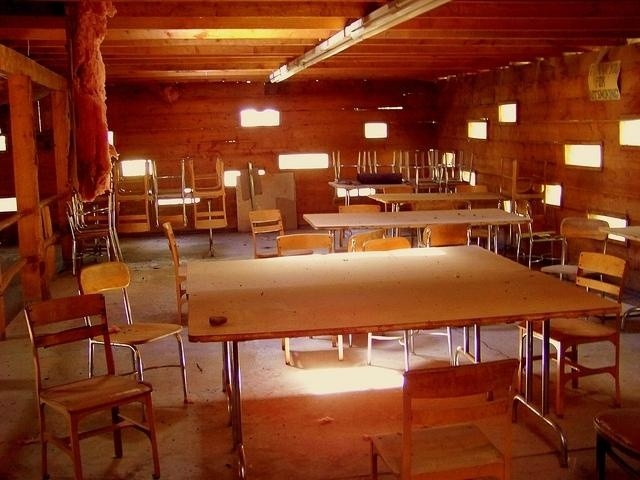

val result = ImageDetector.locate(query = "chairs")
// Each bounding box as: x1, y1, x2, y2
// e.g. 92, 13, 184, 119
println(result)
161, 220, 191, 325
359, 236, 455, 374
26, 149, 639, 254
22, 290, 164, 479
515, 251, 629, 418
74, 260, 192, 426
272, 230, 348, 367
364, 356, 521, 477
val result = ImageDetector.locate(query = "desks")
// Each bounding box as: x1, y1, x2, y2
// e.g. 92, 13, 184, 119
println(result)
177, 243, 625, 476
